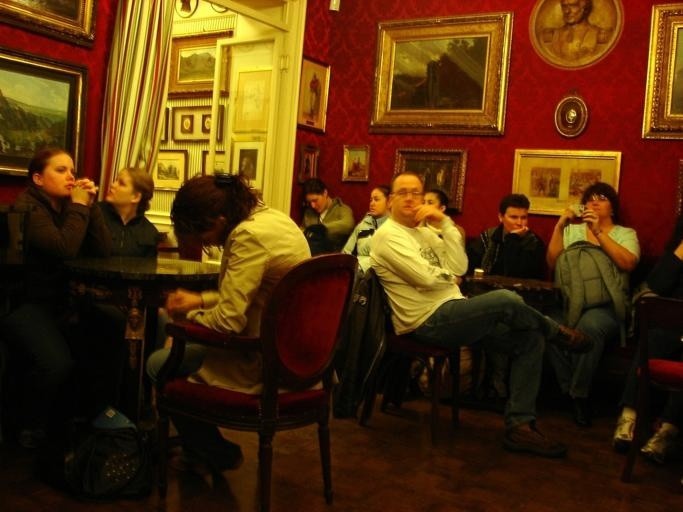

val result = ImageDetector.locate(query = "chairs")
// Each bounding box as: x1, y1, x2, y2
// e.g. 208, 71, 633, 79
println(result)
359, 268, 464, 448
621, 289, 683, 484
154, 254, 358, 512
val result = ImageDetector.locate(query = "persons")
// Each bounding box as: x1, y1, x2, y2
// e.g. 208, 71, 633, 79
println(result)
146, 173, 311, 473
305, 154, 312, 175
341, 183, 392, 306
297, 178, 353, 249
538, 0, 612, 59
88, 168, 159, 267
1, 147, 128, 447
423, 188, 465, 245
613, 203, 683, 461
310, 71, 322, 121
545, 182, 639, 415
368, 173, 595, 458
466, 193, 548, 278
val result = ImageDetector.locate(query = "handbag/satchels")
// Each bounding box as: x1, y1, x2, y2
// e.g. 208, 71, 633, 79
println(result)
54, 427, 152, 502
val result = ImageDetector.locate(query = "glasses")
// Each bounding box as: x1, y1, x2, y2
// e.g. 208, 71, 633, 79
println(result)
588, 194, 608, 201
391, 190, 422, 197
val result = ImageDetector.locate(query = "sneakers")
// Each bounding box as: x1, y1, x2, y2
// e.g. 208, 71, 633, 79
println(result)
548, 323, 593, 354
639, 430, 678, 464
506, 427, 568, 456
613, 417, 637, 454
34, 430, 45, 438
19, 431, 36, 449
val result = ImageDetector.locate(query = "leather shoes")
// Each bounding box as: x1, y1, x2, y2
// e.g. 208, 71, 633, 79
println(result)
573, 404, 592, 427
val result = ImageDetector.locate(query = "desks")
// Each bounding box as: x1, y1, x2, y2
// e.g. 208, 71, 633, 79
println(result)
64, 255, 223, 429
465, 274, 560, 403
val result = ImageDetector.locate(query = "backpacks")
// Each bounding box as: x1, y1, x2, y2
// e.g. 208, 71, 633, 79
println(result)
554, 240, 628, 349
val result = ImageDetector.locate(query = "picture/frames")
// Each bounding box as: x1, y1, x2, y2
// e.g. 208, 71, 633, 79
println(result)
0, 0, 98, 49
367, 11, 514, 138
230, 141, 265, 192
201, 150, 226, 177
159, 107, 169, 146
393, 146, 468, 214
233, 69, 273, 132
511, 148, 622, 219
342, 144, 370, 183
296, 55, 331, 136
151, 149, 188, 191
528, 0, 624, 69
298, 144, 320, 185
167, 31, 233, 101
641, 2, 683, 142
170, 104, 224, 144
1, 47, 89, 185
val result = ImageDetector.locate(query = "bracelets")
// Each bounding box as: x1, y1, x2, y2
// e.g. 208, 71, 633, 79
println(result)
594, 230, 602, 236
